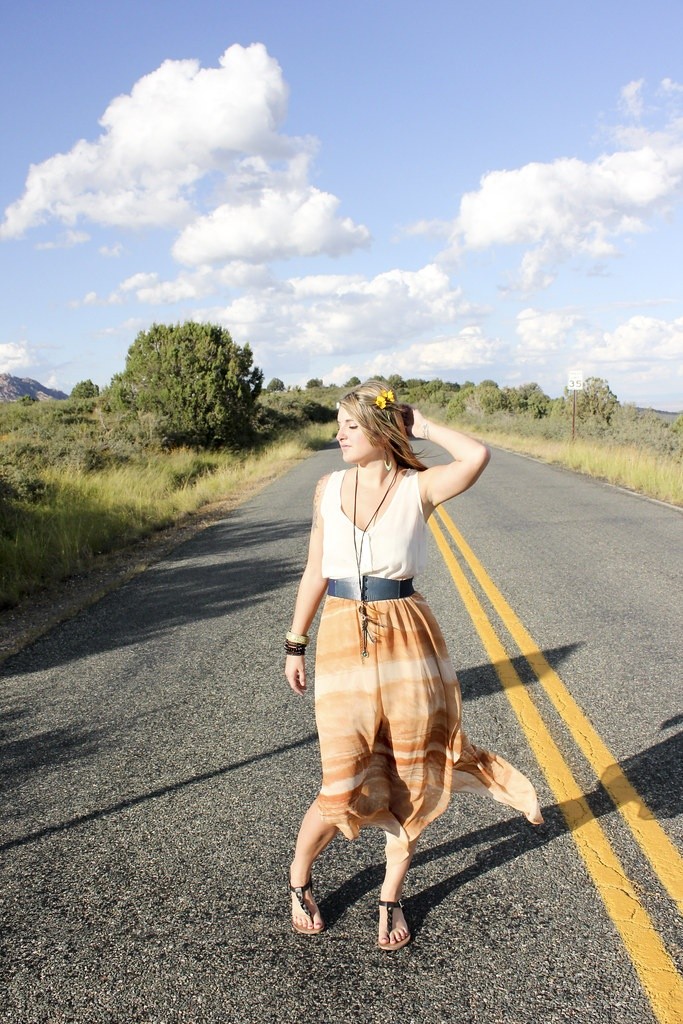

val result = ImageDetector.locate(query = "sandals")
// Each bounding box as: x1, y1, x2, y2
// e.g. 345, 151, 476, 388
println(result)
377, 898, 410, 951
289, 873, 325, 934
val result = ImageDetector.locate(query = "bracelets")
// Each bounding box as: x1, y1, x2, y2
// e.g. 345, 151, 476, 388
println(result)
422, 421, 429, 439
284, 631, 311, 657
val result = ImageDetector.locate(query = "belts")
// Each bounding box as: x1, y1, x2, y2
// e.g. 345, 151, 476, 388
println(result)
326, 575, 415, 603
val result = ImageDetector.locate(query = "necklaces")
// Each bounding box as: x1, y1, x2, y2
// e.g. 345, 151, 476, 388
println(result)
353, 461, 398, 659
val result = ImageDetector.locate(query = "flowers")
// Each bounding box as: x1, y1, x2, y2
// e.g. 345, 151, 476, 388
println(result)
375, 388, 395, 409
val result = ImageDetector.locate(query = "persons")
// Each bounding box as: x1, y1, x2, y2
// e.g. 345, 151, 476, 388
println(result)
284, 378, 543, 952
